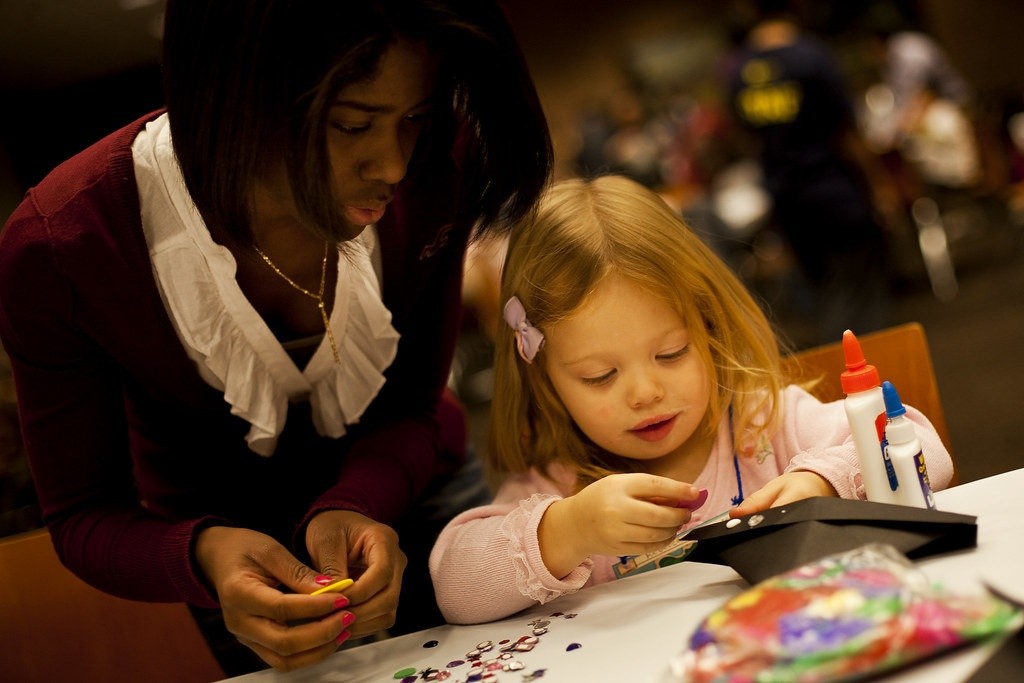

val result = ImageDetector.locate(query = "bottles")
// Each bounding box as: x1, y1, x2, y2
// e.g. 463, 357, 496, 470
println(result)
841, 327, 905, 504
882, 381, 935, 510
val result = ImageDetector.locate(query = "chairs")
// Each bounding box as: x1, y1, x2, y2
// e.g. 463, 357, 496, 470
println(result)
777, 322, 961, 490
0, 524, 228, 683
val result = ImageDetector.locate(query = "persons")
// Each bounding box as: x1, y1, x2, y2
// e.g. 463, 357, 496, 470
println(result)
429, 175, 953, 624
1, 0, 557, 674
579, 0, 1005, 308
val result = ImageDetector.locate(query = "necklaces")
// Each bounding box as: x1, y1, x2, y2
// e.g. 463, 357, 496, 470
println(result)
250, 231, 339, 363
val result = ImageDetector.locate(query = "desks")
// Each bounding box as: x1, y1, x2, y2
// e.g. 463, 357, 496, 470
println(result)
221, 463, 1024, 683
